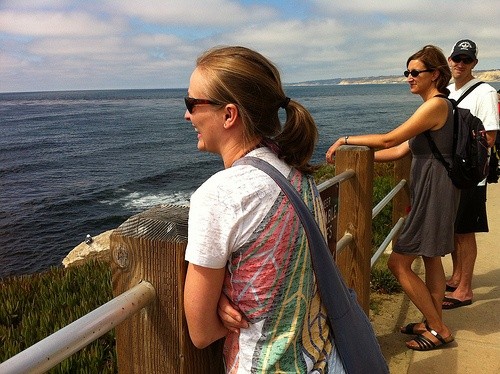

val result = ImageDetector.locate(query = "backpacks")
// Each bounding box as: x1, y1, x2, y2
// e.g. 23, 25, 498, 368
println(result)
426, 94, 488, 187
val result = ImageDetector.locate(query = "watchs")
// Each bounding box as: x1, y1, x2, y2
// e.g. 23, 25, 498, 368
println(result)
346, 135, 349, 145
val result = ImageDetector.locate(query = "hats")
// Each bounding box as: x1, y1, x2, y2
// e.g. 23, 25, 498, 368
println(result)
450, 39, 479, 58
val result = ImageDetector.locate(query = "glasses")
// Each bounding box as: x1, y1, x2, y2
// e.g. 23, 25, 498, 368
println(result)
452, 57, 474, 64
183, 96, 225, 115
403, 70, 435, 78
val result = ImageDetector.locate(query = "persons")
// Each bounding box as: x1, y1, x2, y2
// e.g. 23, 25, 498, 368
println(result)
183, 46, 346, 374
442, 38, 499, 310
326, 44, 460, 351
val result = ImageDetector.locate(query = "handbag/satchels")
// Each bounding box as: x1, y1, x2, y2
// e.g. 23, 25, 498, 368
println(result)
229, 156, 390, 374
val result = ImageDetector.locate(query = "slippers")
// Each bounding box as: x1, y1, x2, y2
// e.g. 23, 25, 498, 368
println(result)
442, 296, 476, 309
445, 285, 457, 292
399, 318, 429, 336
405, 329, 455, 351
